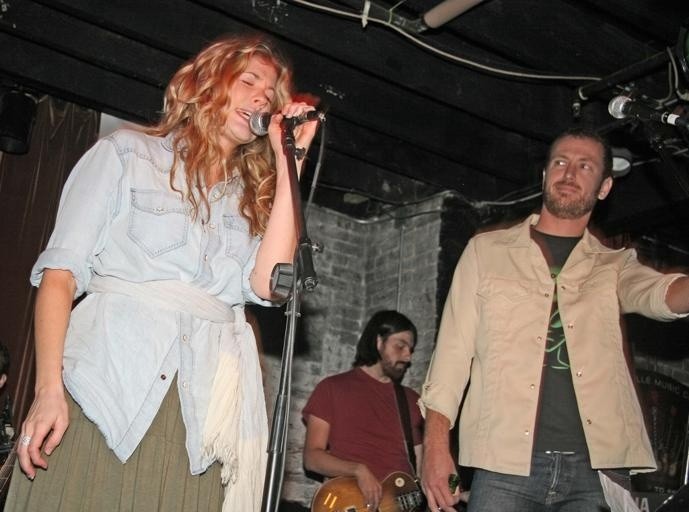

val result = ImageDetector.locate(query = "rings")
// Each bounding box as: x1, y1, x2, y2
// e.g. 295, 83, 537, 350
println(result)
20, 435, 31, 447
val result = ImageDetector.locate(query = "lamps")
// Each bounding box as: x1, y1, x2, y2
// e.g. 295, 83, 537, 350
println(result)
0, 85, 38, 155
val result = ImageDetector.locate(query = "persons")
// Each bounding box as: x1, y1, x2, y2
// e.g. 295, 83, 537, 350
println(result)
300, 308, 470, 511
0, 343, 10, 398
415, 126, 689, 512
4, 35, 319, 512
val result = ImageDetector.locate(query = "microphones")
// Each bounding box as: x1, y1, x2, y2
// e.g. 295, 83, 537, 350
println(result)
248, 110, 322, 136
608, 95, 688, 128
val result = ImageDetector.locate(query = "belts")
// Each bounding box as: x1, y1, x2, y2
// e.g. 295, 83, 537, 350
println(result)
535, 435, 589, 455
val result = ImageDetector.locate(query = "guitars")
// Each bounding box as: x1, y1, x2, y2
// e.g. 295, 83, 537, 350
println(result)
311, 471, 423, 512
